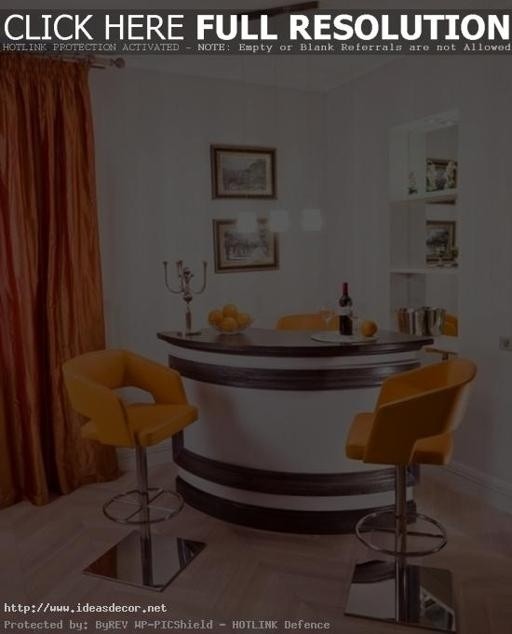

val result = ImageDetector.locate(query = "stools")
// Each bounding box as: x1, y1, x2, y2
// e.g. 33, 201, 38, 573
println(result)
343, 359, 478, 634
62, 349, 206, 593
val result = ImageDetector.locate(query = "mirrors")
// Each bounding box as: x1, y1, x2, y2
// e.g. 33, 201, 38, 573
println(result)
406, 125, 456, 336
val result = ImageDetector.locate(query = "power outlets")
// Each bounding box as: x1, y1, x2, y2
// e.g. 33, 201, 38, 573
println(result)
498, 335, 512, 351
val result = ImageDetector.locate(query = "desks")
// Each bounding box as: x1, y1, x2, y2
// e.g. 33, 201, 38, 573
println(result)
156, 325, 435, 535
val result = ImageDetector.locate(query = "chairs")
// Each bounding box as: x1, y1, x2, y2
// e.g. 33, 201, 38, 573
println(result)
276, 314, 339, 333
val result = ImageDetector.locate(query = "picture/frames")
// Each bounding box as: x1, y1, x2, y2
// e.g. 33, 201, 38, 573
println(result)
209, 143, 277, 201
426, 219, 456, 261
426, 158, 456, 191
212, 217, 281, 274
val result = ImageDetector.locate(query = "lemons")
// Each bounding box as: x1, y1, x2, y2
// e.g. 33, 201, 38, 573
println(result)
361, 321, 378, 336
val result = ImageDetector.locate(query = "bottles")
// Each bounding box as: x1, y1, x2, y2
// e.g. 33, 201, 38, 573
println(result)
335, 280, 355, 335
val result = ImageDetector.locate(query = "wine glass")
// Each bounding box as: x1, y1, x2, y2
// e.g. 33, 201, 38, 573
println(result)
319, 304, 335, 337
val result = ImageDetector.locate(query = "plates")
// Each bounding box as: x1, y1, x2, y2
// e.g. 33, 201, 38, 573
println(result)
214, 327, 255, 337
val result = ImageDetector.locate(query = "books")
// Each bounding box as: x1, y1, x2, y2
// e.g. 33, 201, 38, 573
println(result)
398, 306, 447, 336
